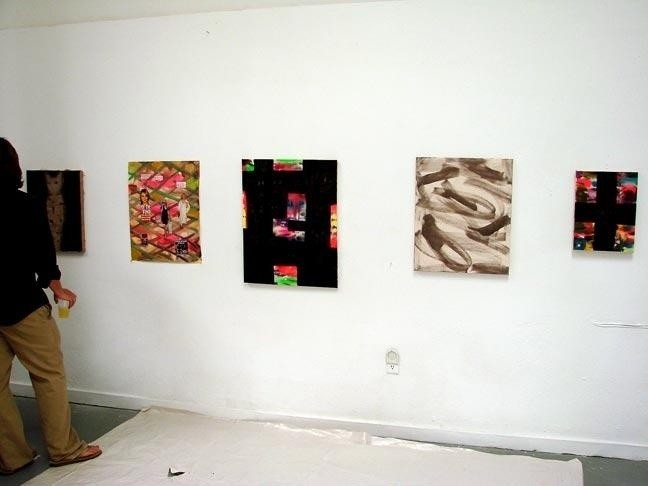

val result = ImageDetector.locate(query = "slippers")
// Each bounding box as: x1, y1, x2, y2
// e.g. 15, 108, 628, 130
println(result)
49, 445, 102, 467
1, 448, 38, 475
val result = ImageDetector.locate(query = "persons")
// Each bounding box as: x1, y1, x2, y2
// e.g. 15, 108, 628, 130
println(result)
1, 136, 102, 475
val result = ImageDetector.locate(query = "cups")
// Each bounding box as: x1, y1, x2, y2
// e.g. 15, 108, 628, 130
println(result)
58, 298, 70, 319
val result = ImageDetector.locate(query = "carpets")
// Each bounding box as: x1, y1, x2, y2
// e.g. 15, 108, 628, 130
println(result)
19, 405, 587, 485
